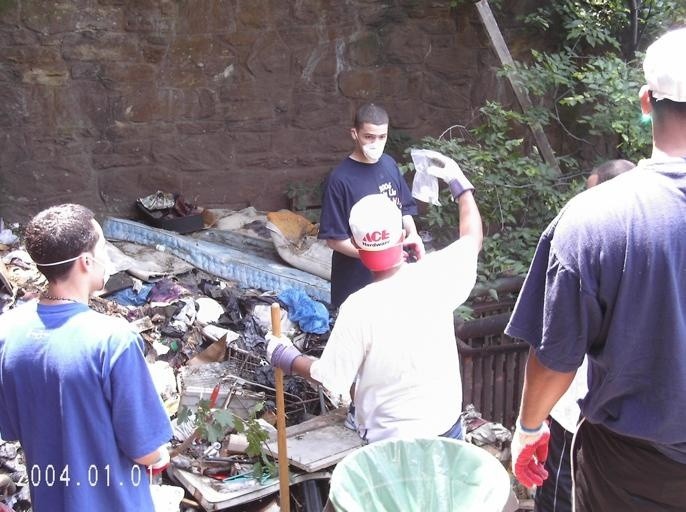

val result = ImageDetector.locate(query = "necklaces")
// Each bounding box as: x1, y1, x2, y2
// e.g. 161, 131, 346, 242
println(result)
34, 292, 78, 307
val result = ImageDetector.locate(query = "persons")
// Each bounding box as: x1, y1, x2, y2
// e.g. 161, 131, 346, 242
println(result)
317, 102, 425, 313
507, 28, 685, 512
529, 157, 638, 512
265, 151, 482, 446
0, 203, 170, 512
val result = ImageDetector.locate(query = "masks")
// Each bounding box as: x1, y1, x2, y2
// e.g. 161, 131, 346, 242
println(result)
356, 137, 386, 162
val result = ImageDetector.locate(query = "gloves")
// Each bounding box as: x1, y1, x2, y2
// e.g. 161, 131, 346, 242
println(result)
264, 331, 303, 376
421, 149, 476, 205
510, 415, 551, 488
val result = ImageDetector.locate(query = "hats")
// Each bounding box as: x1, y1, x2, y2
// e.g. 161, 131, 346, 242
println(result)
643, 28, 686, 102
349, 194, 404, 271
